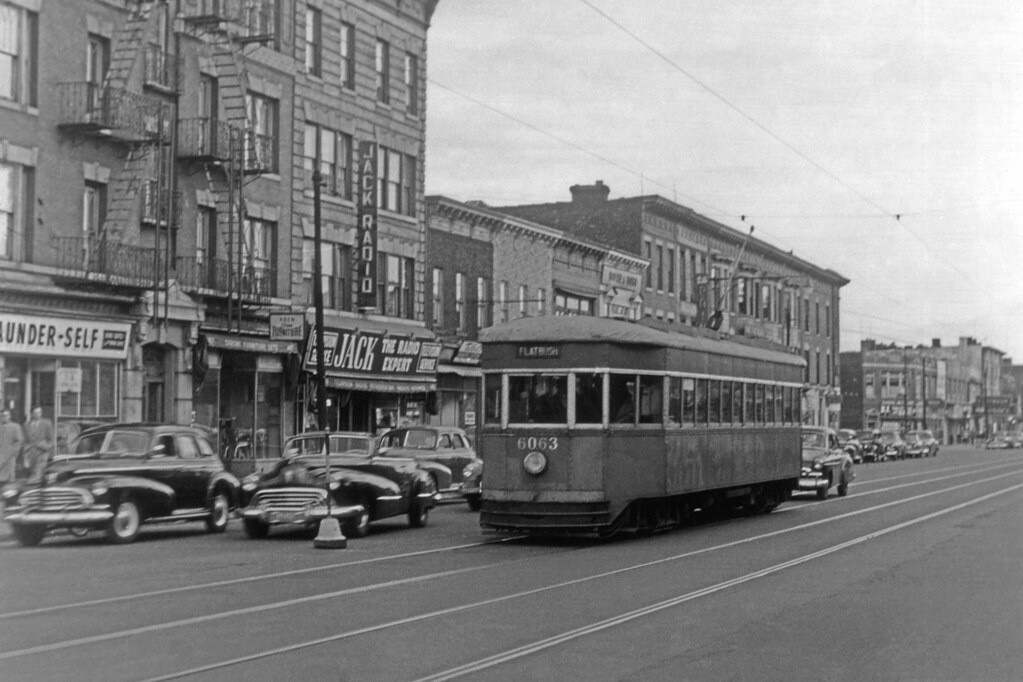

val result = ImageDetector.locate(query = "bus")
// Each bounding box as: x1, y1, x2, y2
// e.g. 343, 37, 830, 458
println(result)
477, 316, 807, 540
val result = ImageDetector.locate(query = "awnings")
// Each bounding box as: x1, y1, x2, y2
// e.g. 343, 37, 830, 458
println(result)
305, 373, 438, 393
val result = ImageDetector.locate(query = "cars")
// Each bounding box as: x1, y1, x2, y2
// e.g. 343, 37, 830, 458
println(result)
903, 430, 939, 457
883, 431, 906, 460
838, 428, 863, 463
2, 422, 241, 543
856, 429, 885, 462
787, 425, 855, 499
376, 425, 483, 509
239, 430, 441, 538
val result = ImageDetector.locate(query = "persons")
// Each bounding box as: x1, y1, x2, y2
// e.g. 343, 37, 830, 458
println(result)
24, 405, 52, 470
533, 376, 622, 424
0, 409, 23, 481
